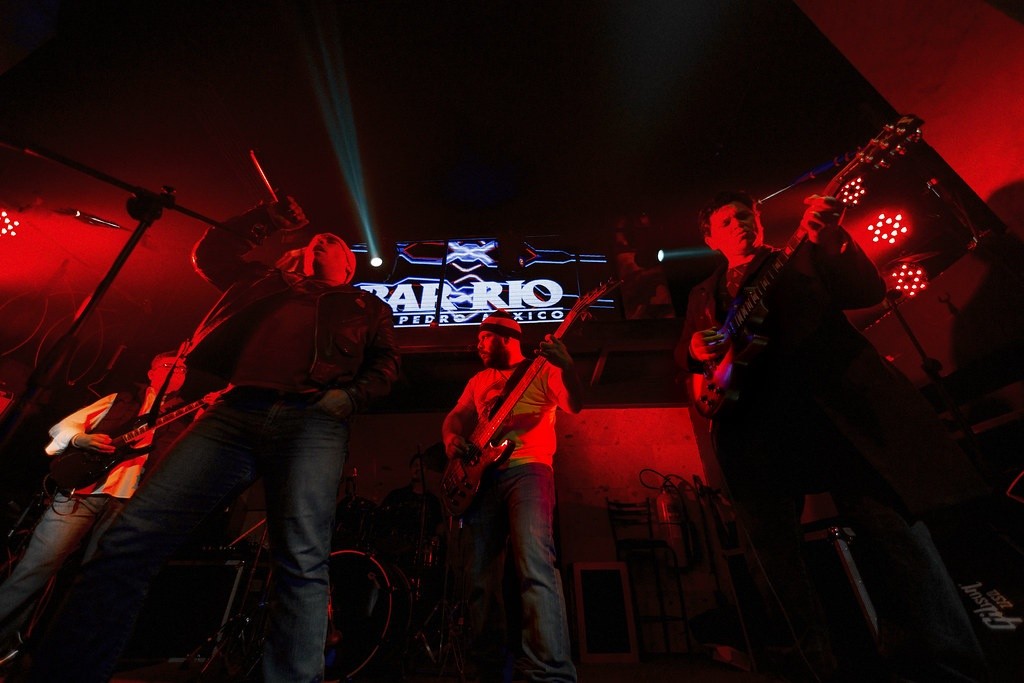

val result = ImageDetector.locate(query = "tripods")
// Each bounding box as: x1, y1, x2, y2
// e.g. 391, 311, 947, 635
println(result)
395, 512, 479, 683
179, 515, 275, 683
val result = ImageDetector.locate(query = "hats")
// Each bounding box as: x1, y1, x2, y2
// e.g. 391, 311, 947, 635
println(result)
479, 308, 522, 340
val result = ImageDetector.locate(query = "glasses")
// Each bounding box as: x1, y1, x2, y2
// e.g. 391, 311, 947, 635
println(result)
152, 363, 187, 374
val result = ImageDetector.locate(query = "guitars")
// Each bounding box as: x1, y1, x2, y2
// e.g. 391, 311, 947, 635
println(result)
425, 273, 625, 519
686, 110, 927, 422
50, 379, 236, 499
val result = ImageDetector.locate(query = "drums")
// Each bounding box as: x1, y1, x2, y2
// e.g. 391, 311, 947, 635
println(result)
323, 548, 415, 683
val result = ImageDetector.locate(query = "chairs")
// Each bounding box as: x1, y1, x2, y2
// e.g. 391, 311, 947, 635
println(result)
606, 498, 695, 662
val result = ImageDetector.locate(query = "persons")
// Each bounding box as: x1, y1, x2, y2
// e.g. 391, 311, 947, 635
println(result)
670, 185, 993, 683
439, 308, 585, 683
0, 187, 445, 683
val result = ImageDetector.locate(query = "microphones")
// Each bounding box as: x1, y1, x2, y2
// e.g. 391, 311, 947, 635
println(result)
416, 443, 423, 457
246, 148, 307, 248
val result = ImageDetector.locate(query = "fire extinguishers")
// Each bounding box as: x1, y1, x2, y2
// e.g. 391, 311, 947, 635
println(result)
656, 480, 695, 570
